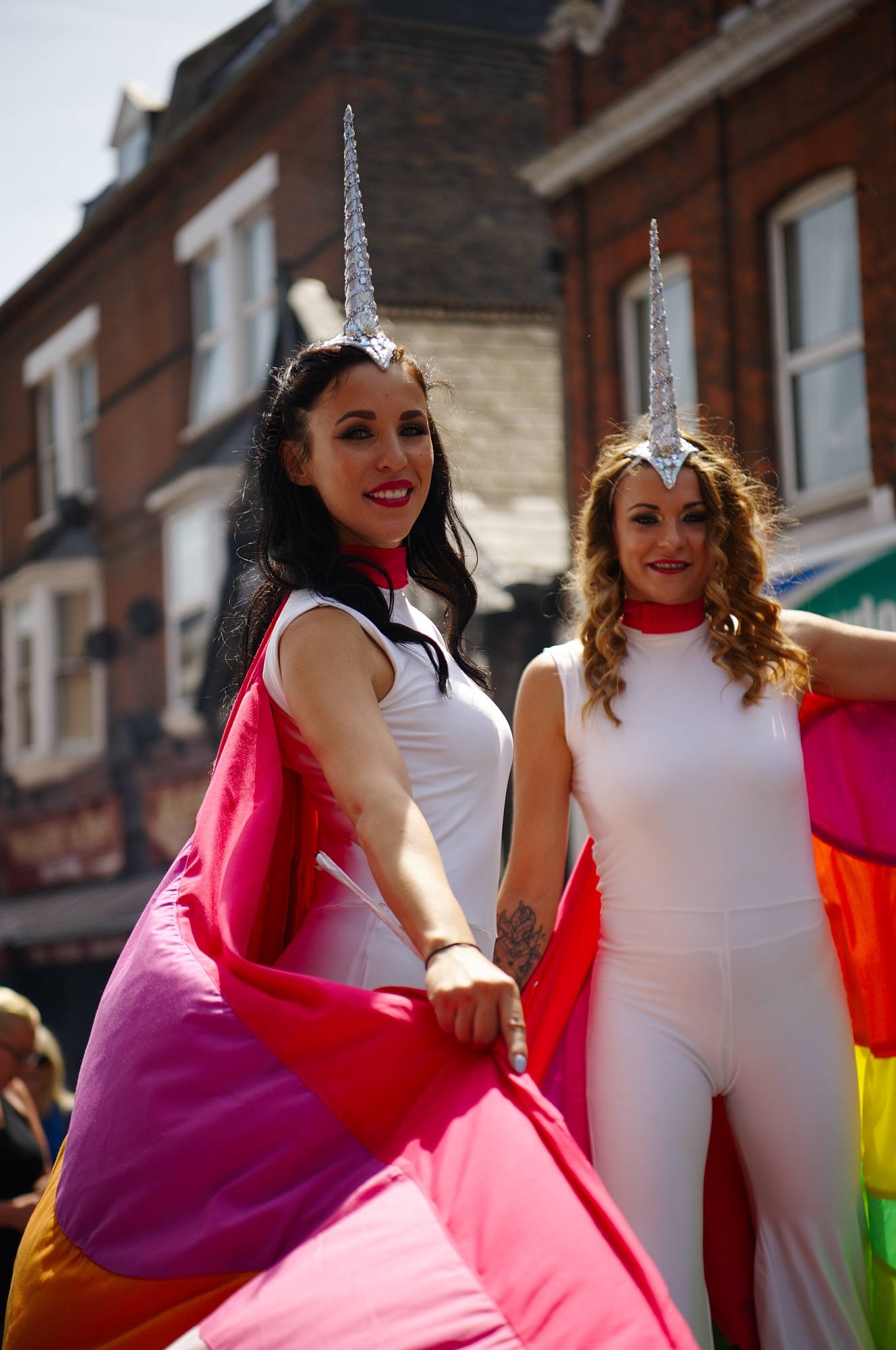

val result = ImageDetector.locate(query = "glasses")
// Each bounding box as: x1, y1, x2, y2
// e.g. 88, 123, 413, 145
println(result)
37, 1055, 52, 1069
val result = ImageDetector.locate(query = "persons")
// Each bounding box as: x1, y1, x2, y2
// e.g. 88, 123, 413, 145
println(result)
492, 409, 896, 1350
193, 334, 529, 1073
0, 987, 78, 1348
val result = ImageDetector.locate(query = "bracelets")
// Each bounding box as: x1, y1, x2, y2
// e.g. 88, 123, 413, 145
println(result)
424, 942, 483, 971
